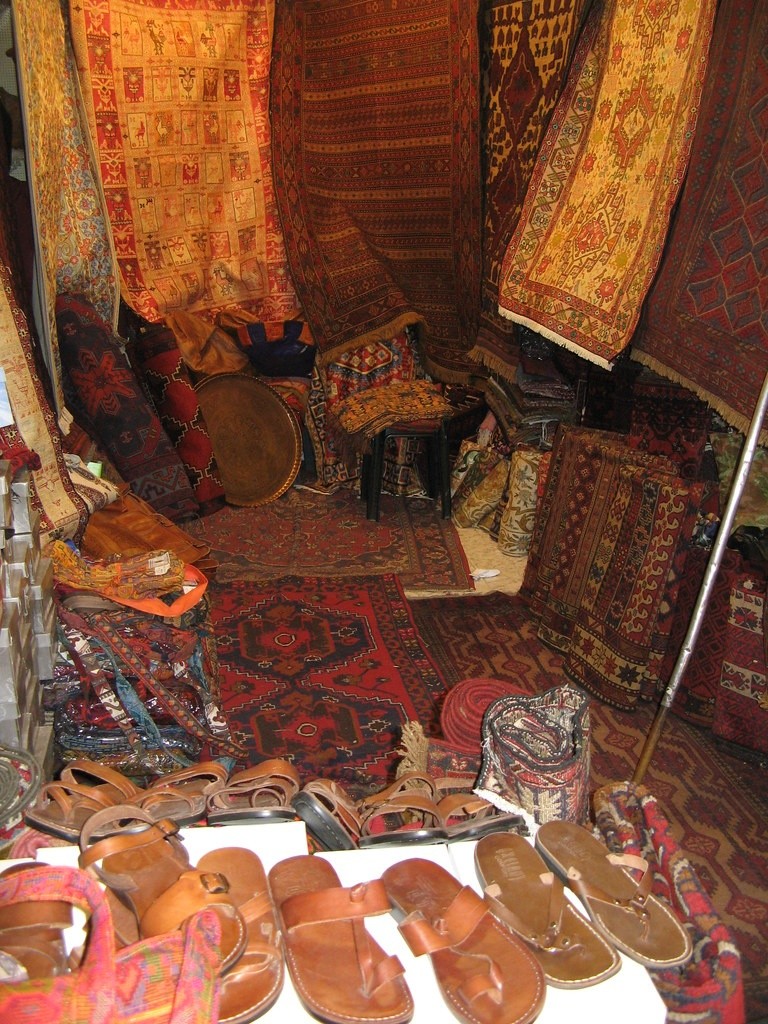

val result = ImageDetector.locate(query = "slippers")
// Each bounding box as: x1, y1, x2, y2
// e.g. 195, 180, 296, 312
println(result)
535, 819, 693, 968
382, 856, 546, 1023
25, 757, 525, 846
268, 858, 416, 1024
196, 846, 284, 1022
474, 831, 623, 990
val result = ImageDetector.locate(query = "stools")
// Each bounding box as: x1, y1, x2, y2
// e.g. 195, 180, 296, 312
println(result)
358, 418, 453, 523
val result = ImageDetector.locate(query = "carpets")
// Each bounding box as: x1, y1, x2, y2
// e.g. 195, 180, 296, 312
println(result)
57, 487, 768, 1023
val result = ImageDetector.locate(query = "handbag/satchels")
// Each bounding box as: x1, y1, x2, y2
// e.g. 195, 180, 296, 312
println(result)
0, 863, 224, 1023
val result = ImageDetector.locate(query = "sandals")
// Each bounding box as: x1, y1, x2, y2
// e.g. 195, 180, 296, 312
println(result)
79, 804, 248, 981
0, 860, 75, 975
